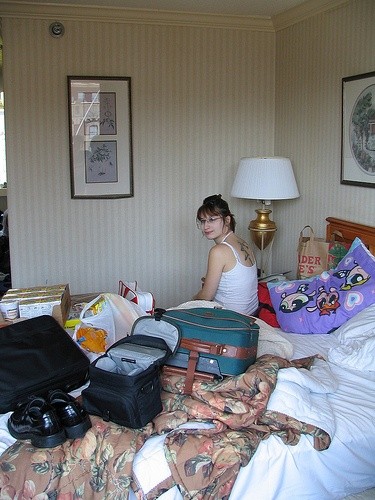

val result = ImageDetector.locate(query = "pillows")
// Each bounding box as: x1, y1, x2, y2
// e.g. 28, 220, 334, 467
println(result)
266, 236, 375, 334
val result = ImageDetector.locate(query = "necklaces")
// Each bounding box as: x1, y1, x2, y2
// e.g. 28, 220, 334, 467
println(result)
219, 231, 233, 245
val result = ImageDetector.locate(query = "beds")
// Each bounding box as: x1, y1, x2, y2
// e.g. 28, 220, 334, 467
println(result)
0, 217, 375, 500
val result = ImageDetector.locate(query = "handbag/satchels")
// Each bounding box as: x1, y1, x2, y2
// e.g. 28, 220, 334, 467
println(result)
72, 293, 149, 355
257, 282, 281, 327
119, 280, 156, 316
0, 315, 92, 414
296, 225, 349, 280
81, 308, 181, 431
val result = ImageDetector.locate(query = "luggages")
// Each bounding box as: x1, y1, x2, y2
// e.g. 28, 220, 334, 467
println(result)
160, 305, 260, 381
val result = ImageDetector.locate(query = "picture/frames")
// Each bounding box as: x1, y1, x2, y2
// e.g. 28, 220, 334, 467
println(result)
67, 75, 134, 199
340, 71, 375, 188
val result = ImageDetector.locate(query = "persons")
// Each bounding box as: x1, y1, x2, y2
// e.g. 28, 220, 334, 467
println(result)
190, 194, 259, 318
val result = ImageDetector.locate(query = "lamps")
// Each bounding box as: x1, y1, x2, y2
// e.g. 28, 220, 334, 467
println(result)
232, 157, 300, 278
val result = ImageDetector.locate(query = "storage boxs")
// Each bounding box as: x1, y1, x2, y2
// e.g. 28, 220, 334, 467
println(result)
0, 284, 111, 331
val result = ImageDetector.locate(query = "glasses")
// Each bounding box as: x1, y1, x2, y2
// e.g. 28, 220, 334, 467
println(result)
200, 218, 221, 225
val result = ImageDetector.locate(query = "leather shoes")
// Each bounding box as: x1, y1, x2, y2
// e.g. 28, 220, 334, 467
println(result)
7, 397, 68, 449
46, 389, 93, 439
